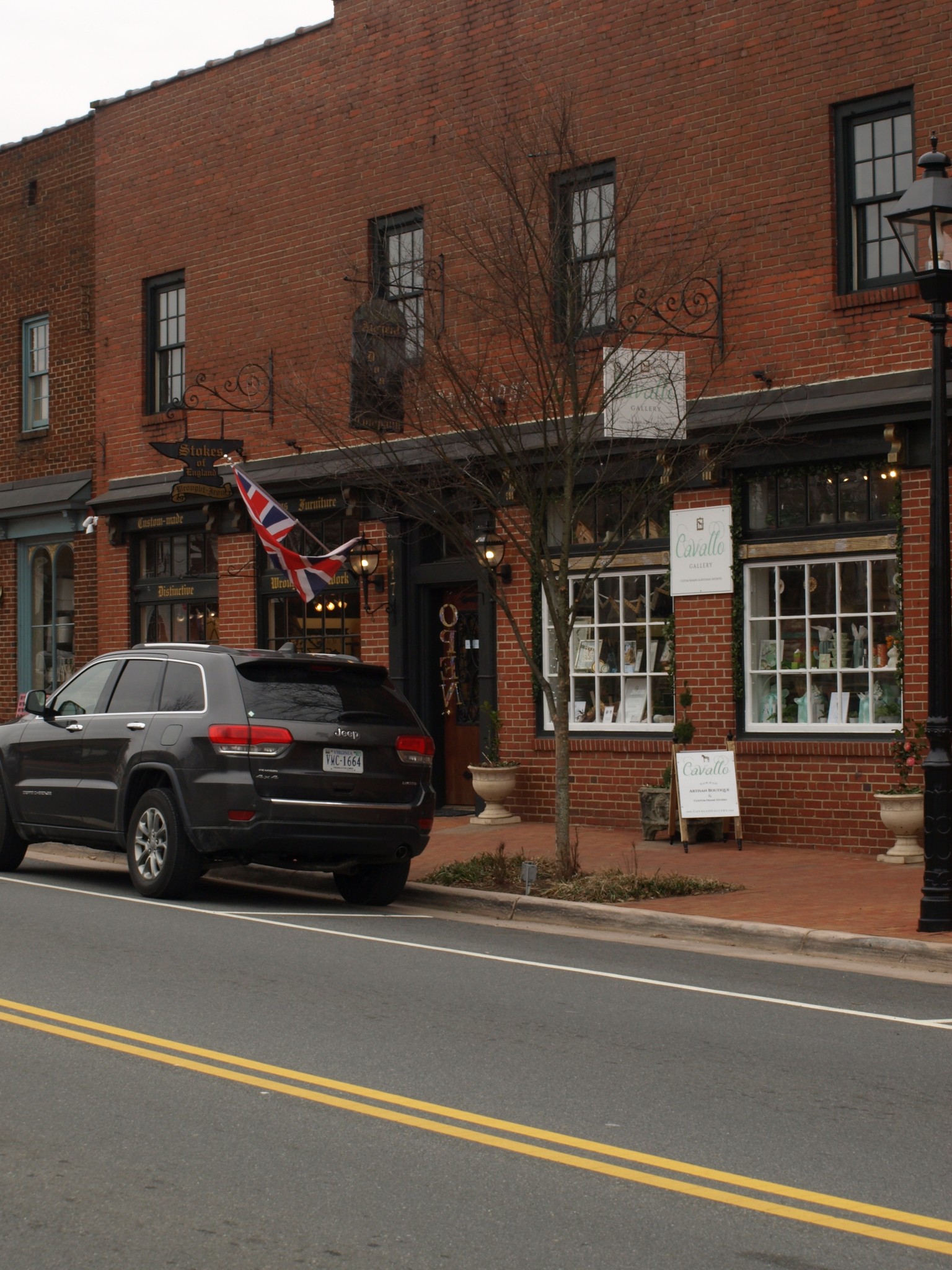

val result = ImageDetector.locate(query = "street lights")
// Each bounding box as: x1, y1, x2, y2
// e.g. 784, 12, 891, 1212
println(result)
883, 127, 951, 933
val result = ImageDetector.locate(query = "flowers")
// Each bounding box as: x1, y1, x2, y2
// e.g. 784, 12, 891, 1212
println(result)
875, 718, 924, 795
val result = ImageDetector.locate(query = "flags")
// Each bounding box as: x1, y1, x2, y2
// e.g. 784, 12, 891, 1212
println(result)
231, 468, 362, 602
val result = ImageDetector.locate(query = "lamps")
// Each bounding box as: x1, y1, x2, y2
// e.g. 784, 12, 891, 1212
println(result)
494, 396, 507, 417
334, 600, 348, 609
313, 596, 322, 612
206, 607, 217, 618
82, 516, 98, 535
474, 520, 512, 589
348, 530, 395, 622
752, 370, 773, 390
176, 610, 184, 621
236, 447, 246, 463
285, 439, 302, 455
196, 608, 204, 619
325, 598, 335, 610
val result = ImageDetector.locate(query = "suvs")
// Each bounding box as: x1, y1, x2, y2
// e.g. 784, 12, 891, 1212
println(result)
1, 642, 436, 908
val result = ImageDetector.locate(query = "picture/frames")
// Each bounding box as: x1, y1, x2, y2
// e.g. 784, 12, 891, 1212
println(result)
575, 639, 604, 670
568, 615, 592, 673
615, 678, 647, 723
758, 639, 785, 670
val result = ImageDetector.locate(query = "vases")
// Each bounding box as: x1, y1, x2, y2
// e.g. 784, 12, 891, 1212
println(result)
875, 792, 925, 865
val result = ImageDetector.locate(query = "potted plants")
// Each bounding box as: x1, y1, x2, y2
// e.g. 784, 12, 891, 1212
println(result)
466, 699, 522, 826
638, 680, 724, 845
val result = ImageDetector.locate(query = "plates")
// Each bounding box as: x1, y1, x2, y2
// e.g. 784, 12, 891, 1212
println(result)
591, 659, 605, 672
600, 664, 610, 673
